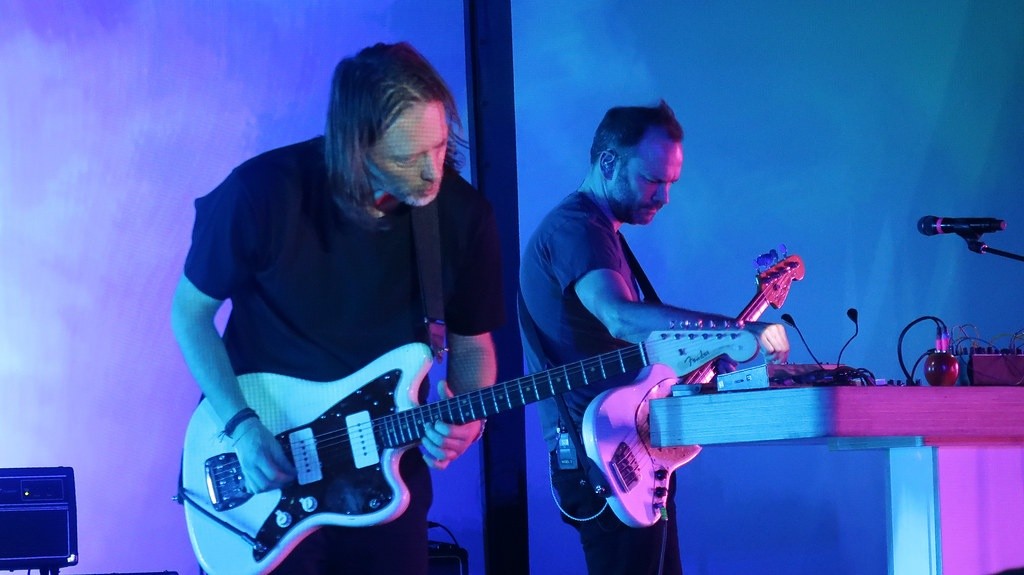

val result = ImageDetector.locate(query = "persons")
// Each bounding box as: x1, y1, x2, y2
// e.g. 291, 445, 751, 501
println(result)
518, 100, 790, 575
166, 39, 495, 575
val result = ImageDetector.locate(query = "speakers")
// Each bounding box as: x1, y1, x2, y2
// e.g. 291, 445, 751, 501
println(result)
428, 541, 469, 575
0, 467, 78, 567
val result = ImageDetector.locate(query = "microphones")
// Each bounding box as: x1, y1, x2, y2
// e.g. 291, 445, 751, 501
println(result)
917, 216, 1007, 242
781, 314, 824, 369
836, 308, 859, 367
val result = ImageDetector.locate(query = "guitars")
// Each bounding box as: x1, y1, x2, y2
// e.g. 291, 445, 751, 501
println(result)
577, 242, 806, 530
180, 318, 760, 575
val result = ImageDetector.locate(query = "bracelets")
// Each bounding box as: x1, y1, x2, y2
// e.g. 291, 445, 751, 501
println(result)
474, 419, 487, 441
217, 408, 263, 447
